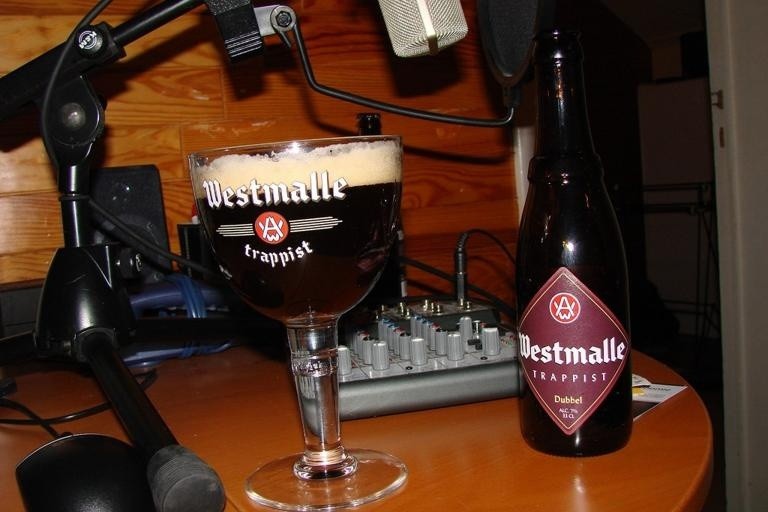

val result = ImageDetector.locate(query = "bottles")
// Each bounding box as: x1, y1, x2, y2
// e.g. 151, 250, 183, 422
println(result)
346, 113, 405, 343
515, 26, 634, 457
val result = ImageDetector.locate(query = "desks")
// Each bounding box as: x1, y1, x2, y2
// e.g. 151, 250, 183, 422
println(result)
0, 347, 716, 512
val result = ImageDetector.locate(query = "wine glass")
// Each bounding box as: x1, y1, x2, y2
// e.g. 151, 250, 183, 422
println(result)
186, 134, 405, 512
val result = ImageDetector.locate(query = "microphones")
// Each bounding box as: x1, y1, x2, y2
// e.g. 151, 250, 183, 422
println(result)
0, 0, 472, 163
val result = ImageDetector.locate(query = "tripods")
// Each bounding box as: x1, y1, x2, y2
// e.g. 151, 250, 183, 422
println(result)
0, 189, 263, 512
